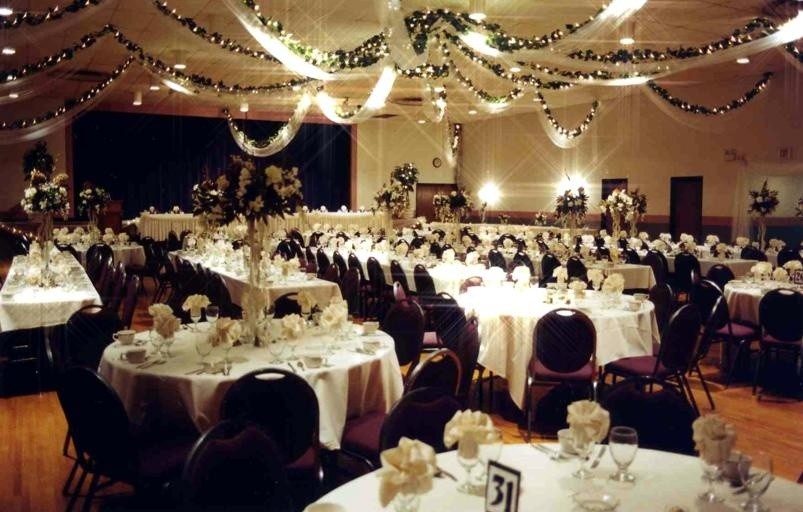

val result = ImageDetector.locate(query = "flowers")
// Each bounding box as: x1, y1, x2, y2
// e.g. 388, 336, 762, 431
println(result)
371, 161, 474, 224
18, 139, 114, 228
190, 151, 306, 226
553, 185, 648, 225
745, 177, 780, 225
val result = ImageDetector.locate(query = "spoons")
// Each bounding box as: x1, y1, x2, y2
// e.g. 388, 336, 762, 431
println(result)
531, 443, 571, 462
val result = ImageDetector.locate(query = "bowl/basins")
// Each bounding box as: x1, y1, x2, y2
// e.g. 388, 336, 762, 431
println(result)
556, 428, 577, 454
718, 452, 751, 481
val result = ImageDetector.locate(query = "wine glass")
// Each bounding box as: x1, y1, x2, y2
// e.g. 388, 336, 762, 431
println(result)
735, 449, 774, 511
696, 455, 726, 502
570, 433, 595, 479
606, 426, 640, 483
146, 302, 354, 361
453, 426, 503, 494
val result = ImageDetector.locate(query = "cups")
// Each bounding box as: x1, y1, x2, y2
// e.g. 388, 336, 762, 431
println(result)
625, 292, 650, 311
567, 490, 620, 510
389, 482, 421, 511
110, 321, 381, 373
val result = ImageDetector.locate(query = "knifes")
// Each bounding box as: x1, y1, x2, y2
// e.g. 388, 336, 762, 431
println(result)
592, 443, 606, 467
436, 467, 457, 482
732, 474, 767, 495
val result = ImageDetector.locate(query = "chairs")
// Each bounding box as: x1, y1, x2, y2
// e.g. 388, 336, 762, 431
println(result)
1, 222, 803, 512
